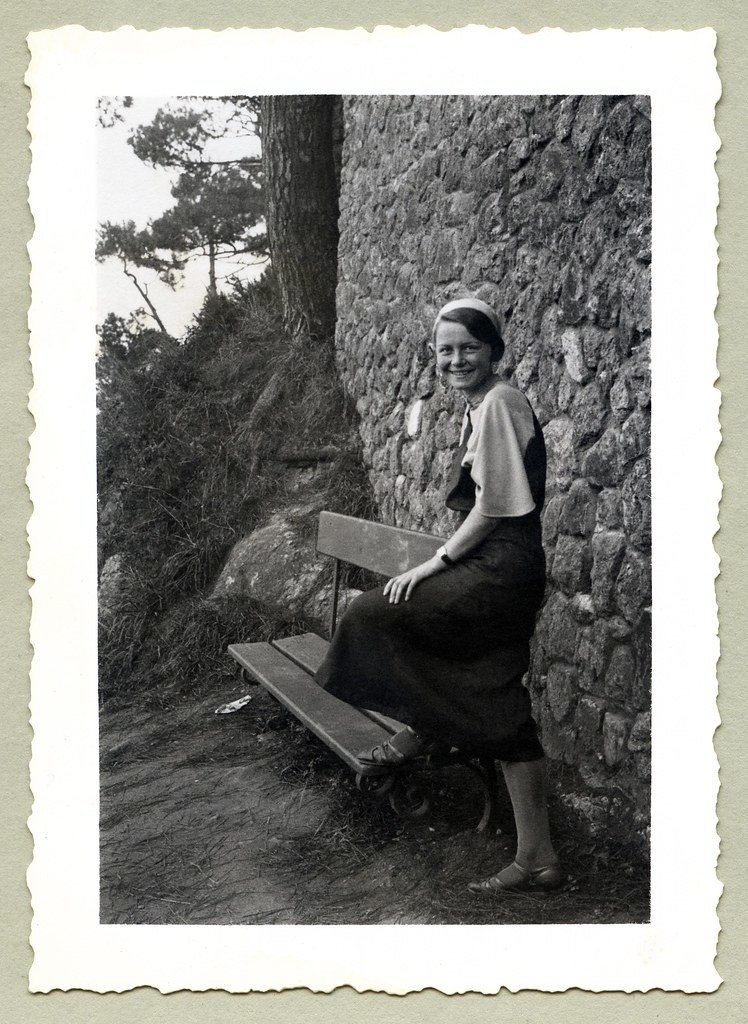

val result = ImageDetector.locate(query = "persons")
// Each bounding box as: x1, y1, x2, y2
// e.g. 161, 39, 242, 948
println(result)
315, 298, 566, 896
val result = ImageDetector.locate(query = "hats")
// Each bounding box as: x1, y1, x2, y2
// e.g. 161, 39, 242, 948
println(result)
432, 298, 501, 345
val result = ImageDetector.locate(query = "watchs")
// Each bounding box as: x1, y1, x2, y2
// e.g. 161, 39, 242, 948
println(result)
436, 545, 454, 566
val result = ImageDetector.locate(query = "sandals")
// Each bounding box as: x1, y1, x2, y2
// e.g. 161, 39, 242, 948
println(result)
356, 725, 453, 768
467, 857, 566, 898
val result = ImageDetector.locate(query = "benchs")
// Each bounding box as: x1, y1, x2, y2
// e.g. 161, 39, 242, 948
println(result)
225, 510, 496, 832
